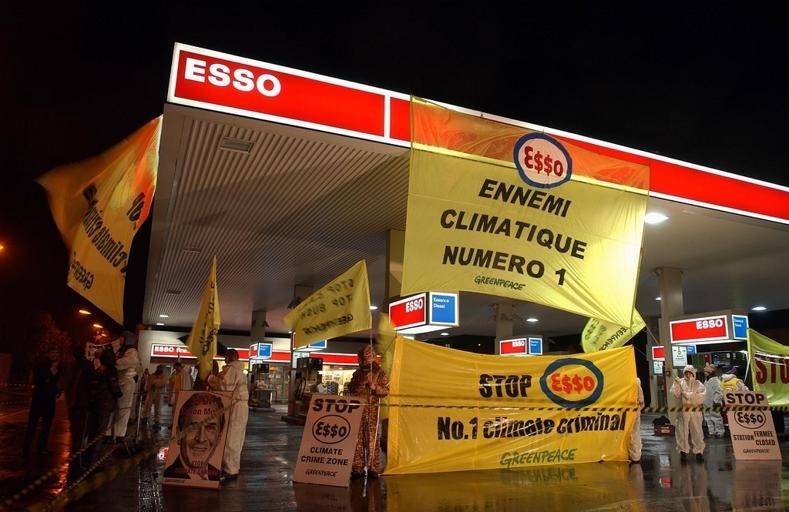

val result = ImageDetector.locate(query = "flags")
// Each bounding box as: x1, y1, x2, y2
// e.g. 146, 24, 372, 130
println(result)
187, 254, 222, 380
580, 305, 647, 353
282, 257, 372, 348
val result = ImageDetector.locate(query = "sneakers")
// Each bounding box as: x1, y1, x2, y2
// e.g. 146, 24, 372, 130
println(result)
223, 470, 237, 481
706, 432, 724, 439
21, 448, 50, 457
680, 452, 688, 462
369, 470, 378, 478
352, 470, 365, 478
105, 436, 126, 445
696, 453, 704, 462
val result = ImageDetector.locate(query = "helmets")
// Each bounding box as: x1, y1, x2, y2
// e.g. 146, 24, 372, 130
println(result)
358, 345, 376, 367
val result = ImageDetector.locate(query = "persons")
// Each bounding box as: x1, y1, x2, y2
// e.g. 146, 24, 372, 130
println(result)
294, 375, 304, 400
626, 374, 646, 464
17, 344, 62, 457
138, 367, 151, 404
667, 363, 706, 463
204, 348, 251, 481
166, 361, 192, 429
59, 344, 96, 463
346, 343, 390, 479
164, 391, 226, 480
87, 347, 124, 434
703, 362, 725, 438
140, 364, 170, 429
719, 361, 751, 392
102, 340, 139, 445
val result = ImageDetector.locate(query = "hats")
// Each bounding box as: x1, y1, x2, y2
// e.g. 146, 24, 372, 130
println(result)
704, 362, 715, 373
722, 365, 736, 374
683, 364, 696, 378
